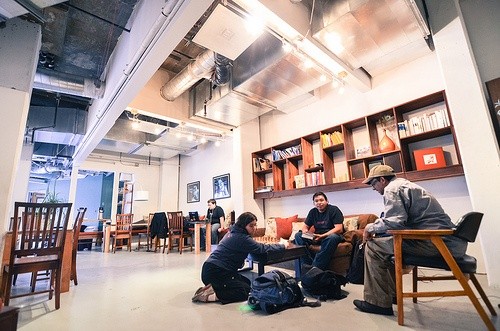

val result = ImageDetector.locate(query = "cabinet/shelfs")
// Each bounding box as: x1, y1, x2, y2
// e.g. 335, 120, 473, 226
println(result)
252, 88, 465, 208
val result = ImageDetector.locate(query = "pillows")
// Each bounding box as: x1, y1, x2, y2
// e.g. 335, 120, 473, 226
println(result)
264, 215, 359, 241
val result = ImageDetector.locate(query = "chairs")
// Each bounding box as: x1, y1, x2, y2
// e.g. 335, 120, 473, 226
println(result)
387, 211, 497, 331
0, 202, 87, 309
112, 211, 192, 254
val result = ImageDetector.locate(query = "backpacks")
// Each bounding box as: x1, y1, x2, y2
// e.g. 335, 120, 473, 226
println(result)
248, 271, 304, 314
346, 242, 365, 284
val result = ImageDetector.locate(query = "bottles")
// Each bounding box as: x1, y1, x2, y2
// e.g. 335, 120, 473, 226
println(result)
379, 129, 395, 154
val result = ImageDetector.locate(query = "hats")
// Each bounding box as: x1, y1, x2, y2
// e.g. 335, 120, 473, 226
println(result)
362, 164, 396, 185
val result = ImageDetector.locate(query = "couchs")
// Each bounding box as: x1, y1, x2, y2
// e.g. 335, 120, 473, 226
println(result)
253, 214, 379, 277
186, 215, 229, 244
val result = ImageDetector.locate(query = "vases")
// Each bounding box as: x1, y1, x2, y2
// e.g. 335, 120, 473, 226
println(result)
378, 129, 395, 153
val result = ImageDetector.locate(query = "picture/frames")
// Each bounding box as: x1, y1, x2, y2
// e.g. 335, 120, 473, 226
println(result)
412, 146, 446, 171
187, 181, 200, 203
212, 173, 231, 199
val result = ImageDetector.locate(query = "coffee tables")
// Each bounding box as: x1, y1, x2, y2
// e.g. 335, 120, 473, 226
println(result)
211, 236, 307, 281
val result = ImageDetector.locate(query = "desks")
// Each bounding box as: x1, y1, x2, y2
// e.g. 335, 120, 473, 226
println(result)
77, 231, 103, 251
0, 229, 74, 299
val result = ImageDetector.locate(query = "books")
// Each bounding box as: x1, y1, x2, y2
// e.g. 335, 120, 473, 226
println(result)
398, 109, 450, 139
322, 131, 344, 148
293, 170, 325, 189
252, 157, 271, 172
301, 231, 316, 241
272, 144, 302, 161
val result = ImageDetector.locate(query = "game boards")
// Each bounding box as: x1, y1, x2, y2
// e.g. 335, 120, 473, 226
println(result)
252, 236, 279, 245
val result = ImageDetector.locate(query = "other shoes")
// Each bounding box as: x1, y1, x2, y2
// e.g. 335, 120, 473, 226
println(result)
192, 284, 213, 303
353, 300, 394, 315
392, 297, 398, 304
200, 247, 206, 251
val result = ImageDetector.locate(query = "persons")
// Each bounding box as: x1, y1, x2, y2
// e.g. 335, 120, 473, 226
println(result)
199, 198, 225, 250
353, 165, 469, 316
191, 212, 291, 302
294, 192, 344, 279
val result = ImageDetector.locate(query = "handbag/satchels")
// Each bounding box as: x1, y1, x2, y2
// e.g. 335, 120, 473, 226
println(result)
301, 266, 345, 300
